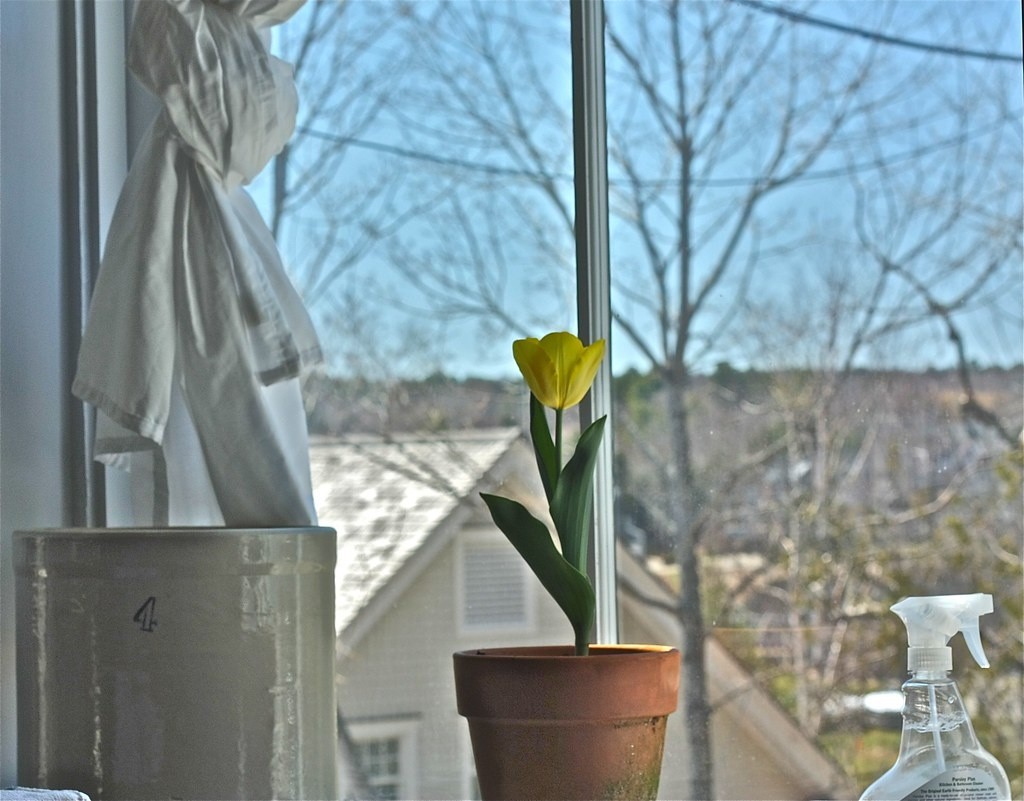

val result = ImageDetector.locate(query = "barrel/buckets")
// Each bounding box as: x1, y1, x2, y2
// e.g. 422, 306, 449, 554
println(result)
11, 527, 337, 801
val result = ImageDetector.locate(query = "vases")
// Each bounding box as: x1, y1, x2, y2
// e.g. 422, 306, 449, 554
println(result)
454, 644, 683, 800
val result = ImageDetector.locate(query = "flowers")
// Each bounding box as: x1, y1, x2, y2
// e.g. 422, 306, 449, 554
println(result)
478, 332, 608, 656
